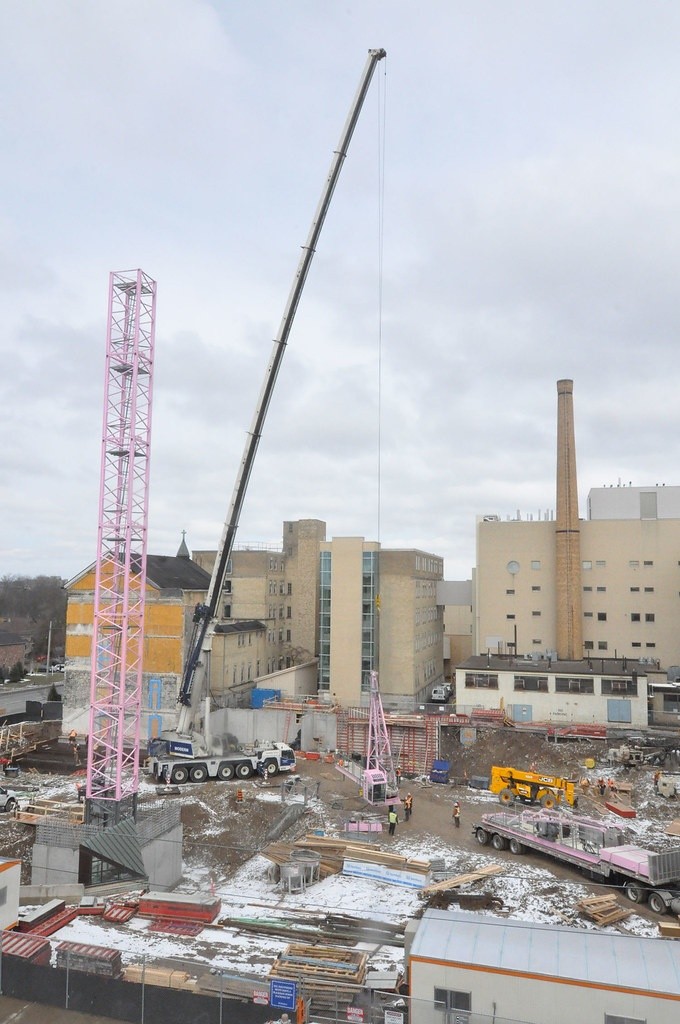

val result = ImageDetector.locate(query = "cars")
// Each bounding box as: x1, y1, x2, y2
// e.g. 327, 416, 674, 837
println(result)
37, 655, 64, 674
442, 682, 455, 695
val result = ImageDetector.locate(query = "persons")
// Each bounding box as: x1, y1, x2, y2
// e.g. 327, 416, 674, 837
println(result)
254, 739, 259, 748
452, 800, 460, 828
597, 777, 619, 796
654, 769, 660, 785
0, 757, 8, 771
83, 735, 89, 761
389, 808, 399, 836
72, 744, 82, 766
401, 792, 413, 821
68, 729, 77, 744
396, 768, 401, 784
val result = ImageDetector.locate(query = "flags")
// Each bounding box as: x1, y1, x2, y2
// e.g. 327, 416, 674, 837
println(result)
375, 594, 381, 611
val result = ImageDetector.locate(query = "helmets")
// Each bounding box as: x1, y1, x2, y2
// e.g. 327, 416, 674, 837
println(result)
407, 792, 411, 796
397, 765, 400, 768
454, 803, 458, 807
455, 800, 459, 802
393, 809, 397, 812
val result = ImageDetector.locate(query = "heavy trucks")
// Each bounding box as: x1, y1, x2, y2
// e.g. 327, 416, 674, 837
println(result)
147, 741, 298, 785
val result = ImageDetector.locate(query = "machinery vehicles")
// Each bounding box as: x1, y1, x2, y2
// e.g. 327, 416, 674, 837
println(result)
491, 765, 579, 810
146, 45, 387, 765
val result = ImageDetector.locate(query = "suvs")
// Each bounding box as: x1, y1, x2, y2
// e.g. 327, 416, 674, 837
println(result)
433, 686, 450, 704
0, 786, 16, 813
